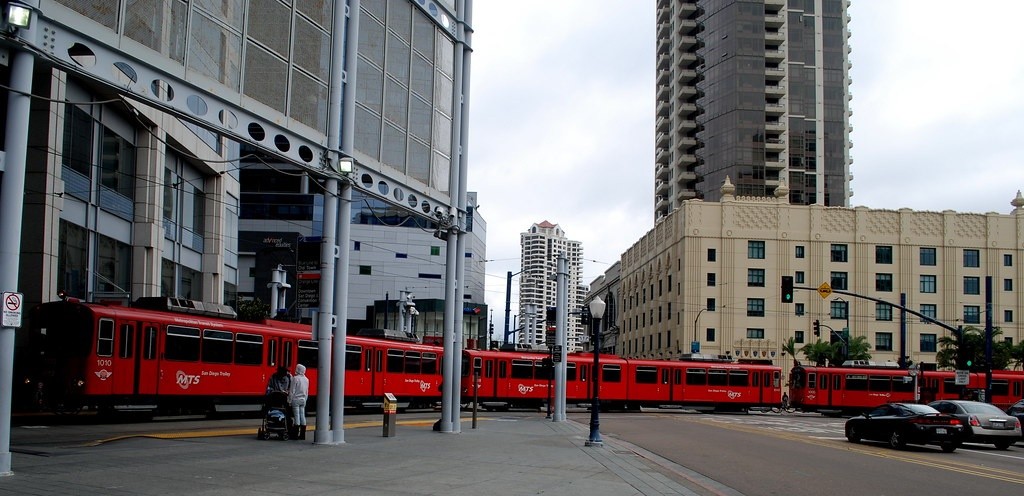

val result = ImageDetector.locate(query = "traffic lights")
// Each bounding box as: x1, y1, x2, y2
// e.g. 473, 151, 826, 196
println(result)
781, 275, 794, 303
964, 346, 974, 370
490, 323, 494, 334
813, 320, 820, 337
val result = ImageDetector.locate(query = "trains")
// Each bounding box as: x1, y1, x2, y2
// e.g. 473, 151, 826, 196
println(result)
13, 294, 1024, 414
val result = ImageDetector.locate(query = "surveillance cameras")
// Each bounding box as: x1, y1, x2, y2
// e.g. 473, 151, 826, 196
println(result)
449, 207, 457, 218
407, 292, 412, 299
436, 207, 443, 214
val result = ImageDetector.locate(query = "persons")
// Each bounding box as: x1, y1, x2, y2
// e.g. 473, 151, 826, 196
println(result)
266, 364, 309, 439
782, 392, 788, 410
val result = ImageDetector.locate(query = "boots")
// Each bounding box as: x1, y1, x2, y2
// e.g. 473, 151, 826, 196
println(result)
298, 423, 307, 440
288, 424, 300, 440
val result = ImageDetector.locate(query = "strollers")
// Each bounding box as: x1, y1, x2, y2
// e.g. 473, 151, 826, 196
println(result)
257, 392, 291, 440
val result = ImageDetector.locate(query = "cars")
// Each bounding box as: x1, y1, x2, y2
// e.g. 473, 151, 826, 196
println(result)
926, 399, 1024, 449
844, 403, 968, 453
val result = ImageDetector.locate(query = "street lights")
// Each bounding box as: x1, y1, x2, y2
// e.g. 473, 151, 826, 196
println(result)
504, 265, 538, 344
585, 295, 606, 447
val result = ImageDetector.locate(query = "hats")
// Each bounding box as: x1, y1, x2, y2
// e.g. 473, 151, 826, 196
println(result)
295, 364, 306, 375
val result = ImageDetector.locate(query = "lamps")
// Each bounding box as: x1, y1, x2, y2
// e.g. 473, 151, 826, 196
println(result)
6, 1, 34, 35
339, 157, 354, 176
